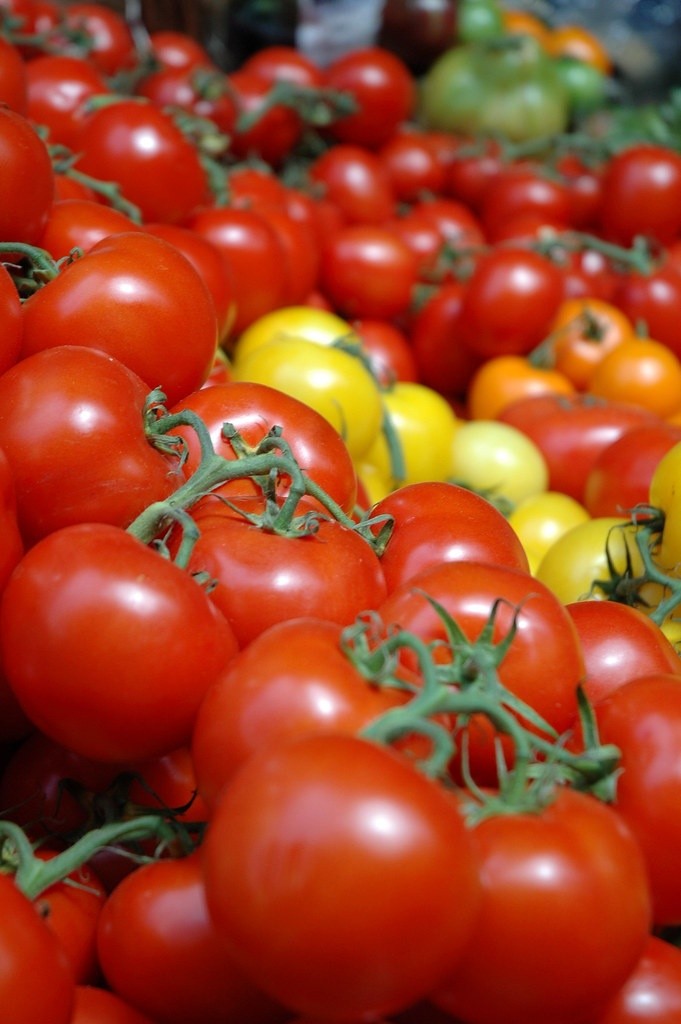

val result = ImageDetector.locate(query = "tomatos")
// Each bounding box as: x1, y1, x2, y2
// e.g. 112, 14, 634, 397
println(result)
0, 0, 681, 1024
